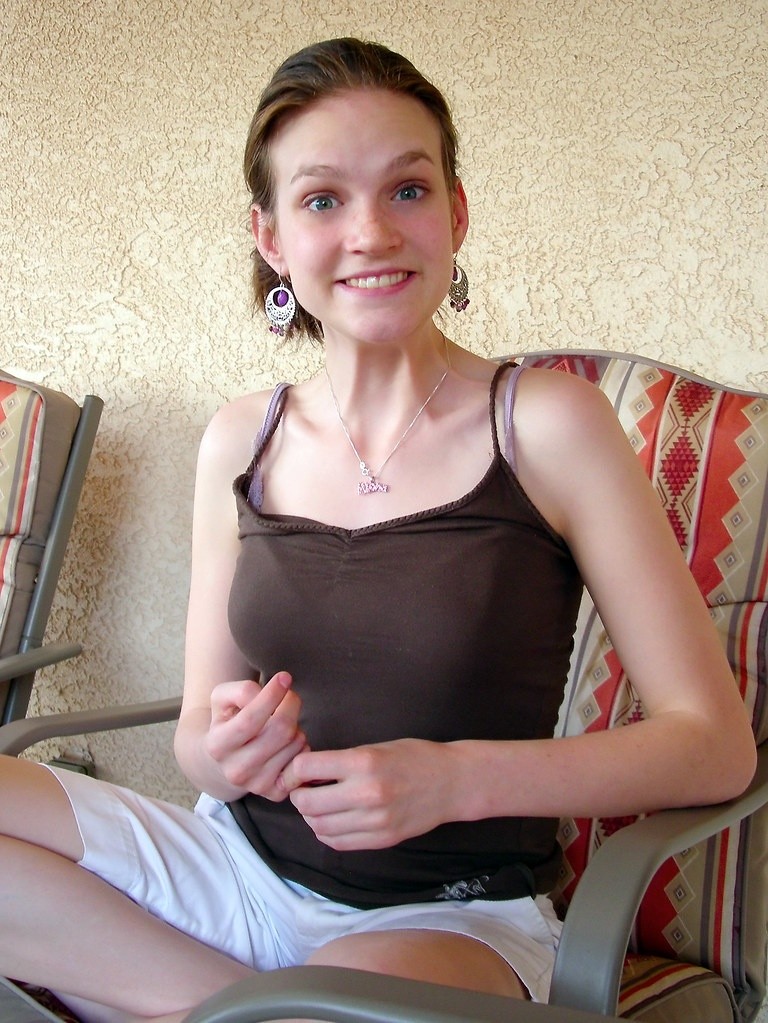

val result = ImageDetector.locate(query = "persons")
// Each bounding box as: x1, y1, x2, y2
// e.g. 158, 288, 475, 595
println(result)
0, 37, 756, 1023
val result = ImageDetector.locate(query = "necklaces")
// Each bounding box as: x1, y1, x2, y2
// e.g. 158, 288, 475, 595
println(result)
323, 327, 451, 496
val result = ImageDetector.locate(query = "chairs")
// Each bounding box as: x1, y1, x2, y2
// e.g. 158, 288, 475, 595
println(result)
0, 370, 105, 728
0, 349, 768, 1023
